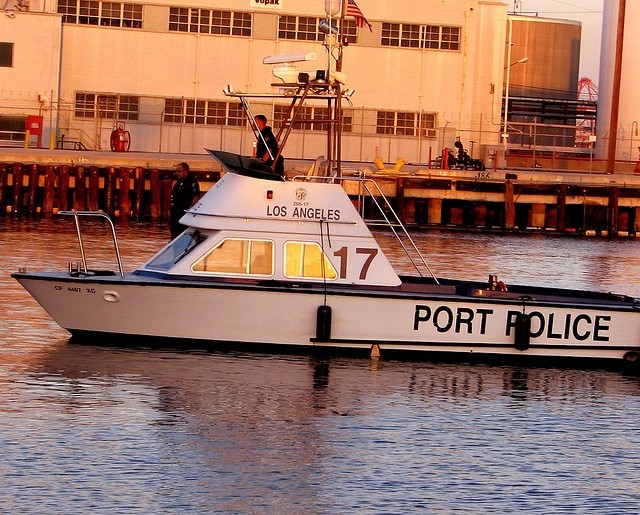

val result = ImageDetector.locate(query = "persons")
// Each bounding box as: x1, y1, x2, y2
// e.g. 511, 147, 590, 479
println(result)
168, 162, 200, 240
252, 114, 284, 180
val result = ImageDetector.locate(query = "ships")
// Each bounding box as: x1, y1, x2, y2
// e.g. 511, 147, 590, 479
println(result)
11, 0, 640, 362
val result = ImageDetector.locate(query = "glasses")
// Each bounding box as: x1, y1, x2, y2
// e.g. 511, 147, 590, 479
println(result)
176, 170, 185, 172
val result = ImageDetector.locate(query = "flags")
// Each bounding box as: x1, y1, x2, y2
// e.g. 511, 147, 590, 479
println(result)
345, 0, 373, 33
